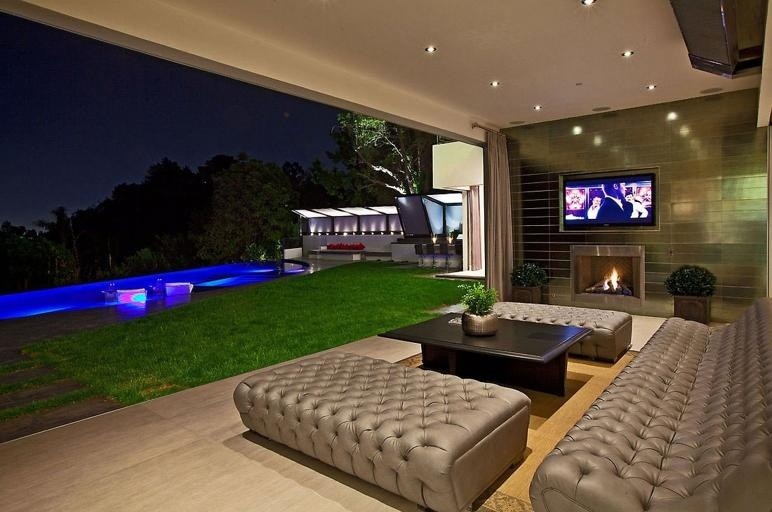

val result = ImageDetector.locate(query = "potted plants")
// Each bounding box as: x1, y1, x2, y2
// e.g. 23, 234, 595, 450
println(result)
509, 263, 549, 303
456, 281, 500, 337
663, 265, 717, 324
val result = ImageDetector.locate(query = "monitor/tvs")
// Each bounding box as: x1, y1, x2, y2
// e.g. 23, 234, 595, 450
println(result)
563, 173, 656, 231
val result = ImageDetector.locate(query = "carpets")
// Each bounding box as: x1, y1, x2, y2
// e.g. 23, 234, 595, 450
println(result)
528, 295, 772, 511
386, 348, 639, 512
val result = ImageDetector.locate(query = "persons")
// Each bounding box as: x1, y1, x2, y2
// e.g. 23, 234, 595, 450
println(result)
588, 182, 648, 220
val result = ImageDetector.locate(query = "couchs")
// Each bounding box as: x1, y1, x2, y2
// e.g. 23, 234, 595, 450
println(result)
232, 350, 532, 512
456, 299, 632, 363
